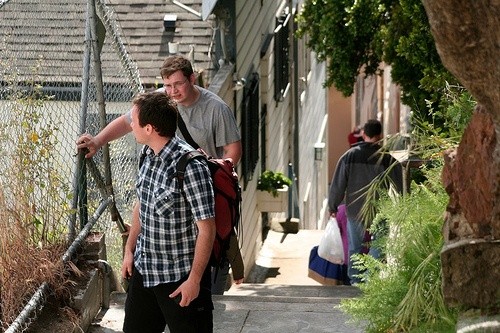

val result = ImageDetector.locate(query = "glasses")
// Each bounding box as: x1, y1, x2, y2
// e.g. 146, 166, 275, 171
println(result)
163, 79, 187, 90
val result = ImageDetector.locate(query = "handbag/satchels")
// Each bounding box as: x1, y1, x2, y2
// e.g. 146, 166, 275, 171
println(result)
318, 215, 344, 265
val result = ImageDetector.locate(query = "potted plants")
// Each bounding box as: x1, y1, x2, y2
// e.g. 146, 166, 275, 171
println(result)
256, 170, 293, 213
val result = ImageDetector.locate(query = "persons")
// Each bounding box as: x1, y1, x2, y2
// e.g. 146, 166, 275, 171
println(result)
75, 55, 245, 294
329, 120, 404, 287
121, 91, 217, 333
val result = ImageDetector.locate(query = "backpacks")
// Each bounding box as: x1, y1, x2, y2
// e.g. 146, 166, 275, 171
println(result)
140, 145, 239, 267
